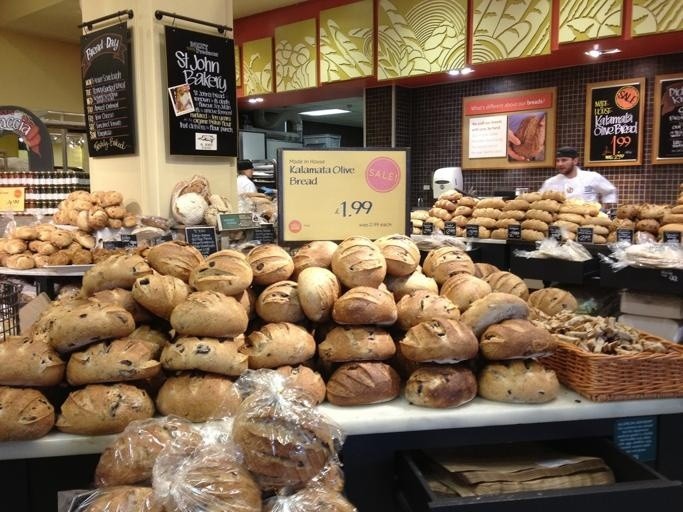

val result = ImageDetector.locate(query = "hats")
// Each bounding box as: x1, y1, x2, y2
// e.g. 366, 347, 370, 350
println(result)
555, 146, 579, 158
237, 160, 254, 171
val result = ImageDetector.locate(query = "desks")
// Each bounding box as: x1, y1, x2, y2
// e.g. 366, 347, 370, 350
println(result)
0, 264, 93, 297
2, 384, 683, 512
410, 239, 683, 301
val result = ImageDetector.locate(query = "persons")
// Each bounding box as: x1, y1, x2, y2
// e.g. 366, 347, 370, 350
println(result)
538, 147, 617, 211
236, 159, 267, 213
507, 131, 526, 162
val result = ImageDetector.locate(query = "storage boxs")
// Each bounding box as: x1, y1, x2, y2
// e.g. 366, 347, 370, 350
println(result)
612, 415, 658, 464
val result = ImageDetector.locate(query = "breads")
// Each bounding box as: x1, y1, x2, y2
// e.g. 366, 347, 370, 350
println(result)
0, 189, 145, 268
405, 190, 683, 243
0, 241, 577, 440
511, 116, 545, 159
78, 388, 350, 511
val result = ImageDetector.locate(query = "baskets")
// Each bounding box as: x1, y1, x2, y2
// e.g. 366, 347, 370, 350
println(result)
535, 319, 683, 404
0, 279, 23, 345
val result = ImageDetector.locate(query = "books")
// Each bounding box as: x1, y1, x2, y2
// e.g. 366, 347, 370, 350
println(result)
432, 451, 617, 497
